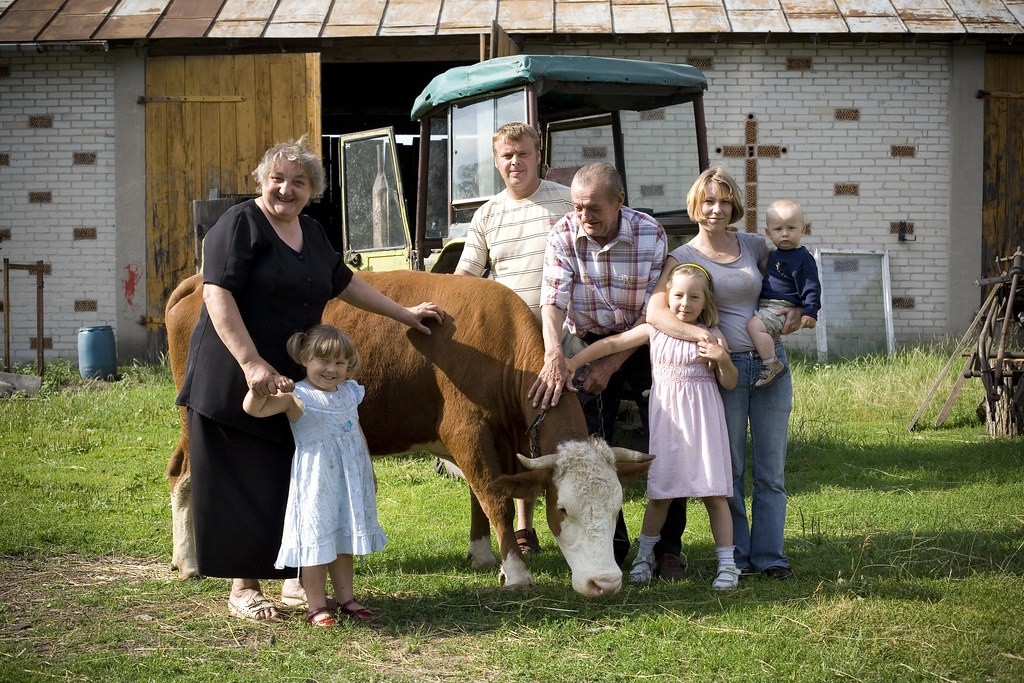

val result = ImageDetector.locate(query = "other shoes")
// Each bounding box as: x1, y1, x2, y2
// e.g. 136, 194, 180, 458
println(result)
659, 553, 682, 579
736, 561, 753, 579
763, 566, 794, 581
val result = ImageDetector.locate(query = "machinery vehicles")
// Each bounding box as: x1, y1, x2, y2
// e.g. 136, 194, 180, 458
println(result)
338, 53, 707, 280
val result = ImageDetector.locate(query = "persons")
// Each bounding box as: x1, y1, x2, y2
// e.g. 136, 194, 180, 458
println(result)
644, 166, 794, 580
564, 264, 741, 591
523, 161, 688, 580
453, 122, 576, 558
747, 198, 821, 390
173, 137, 445, 622
241, 324, 386, 628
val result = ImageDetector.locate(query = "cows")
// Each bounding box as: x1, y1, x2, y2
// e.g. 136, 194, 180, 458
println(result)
161, 266, 658, 604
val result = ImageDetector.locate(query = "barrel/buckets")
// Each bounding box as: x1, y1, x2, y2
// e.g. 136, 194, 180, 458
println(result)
77, 326, 117, 380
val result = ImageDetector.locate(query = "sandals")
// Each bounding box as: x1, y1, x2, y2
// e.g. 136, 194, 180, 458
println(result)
630, 556, 658, 581
712, 566, 741, 591
337, 598, 379, 619
754, 359, 788, 390
306, 607, 335, 627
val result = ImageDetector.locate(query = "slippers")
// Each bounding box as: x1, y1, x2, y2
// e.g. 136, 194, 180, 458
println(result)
281, 593, 309, 609
228, 593, 279, 624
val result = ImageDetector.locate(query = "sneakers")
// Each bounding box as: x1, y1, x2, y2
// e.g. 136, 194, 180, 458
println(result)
614, 541, 630, 567
514, 528, 538, 556
